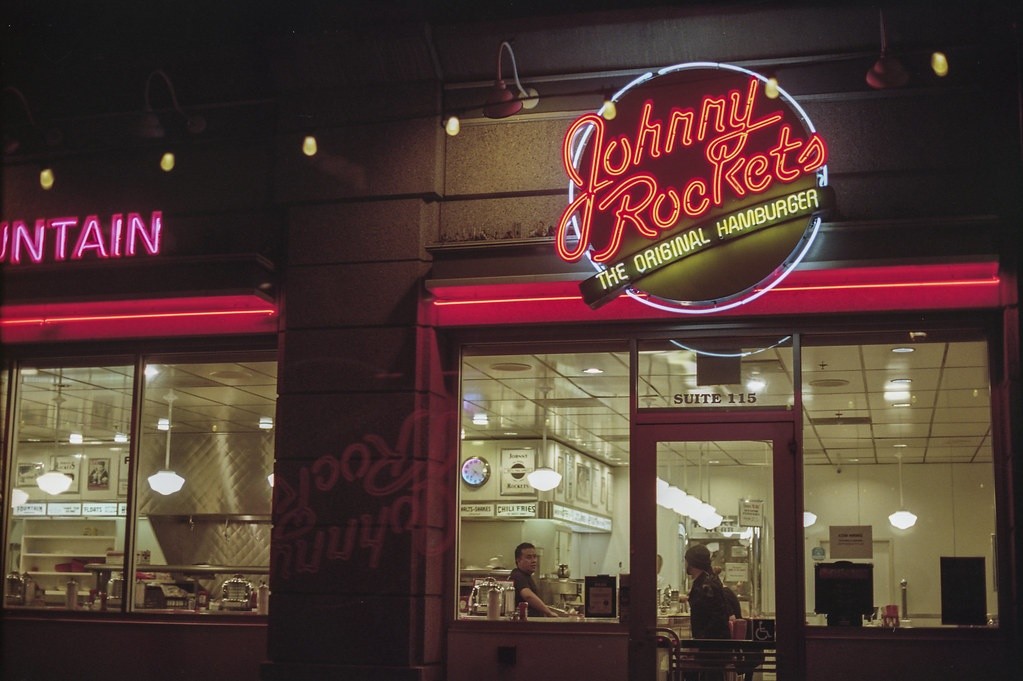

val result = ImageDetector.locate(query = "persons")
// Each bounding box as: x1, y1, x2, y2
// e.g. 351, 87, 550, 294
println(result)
508, 543, 558, 617
685, 545, 731, 638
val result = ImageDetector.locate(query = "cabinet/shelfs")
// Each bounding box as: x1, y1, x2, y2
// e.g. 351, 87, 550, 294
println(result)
20, 519, 117, 601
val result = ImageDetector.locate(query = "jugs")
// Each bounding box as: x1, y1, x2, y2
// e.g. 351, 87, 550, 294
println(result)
558, 563, 569, 578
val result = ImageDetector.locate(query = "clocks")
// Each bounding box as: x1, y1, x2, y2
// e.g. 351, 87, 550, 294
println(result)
460, 455, 493, 488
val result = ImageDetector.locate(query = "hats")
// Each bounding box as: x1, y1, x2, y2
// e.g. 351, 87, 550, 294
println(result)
685, 546, 710, 570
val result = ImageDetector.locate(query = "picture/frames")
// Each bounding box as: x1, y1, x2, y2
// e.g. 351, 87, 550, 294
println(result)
14, 461, 43, 486
48, 453, 82, 494
86, 457, 111, 491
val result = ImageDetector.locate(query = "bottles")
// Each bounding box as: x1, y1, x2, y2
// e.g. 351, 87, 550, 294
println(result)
518, 599, 528, 621
5, 570, 22, 605
66, 578, 78, 611
256, 583, 269, 616
198, 591, 208, 611
488, 586, 502, 619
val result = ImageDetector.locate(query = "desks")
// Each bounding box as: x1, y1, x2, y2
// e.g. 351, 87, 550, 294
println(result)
85, 560, 273, 615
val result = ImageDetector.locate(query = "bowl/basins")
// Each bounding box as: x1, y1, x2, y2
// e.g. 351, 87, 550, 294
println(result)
56, 559, 101, 574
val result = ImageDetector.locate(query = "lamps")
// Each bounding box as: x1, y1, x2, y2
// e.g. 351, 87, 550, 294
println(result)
864, 6, 911, 89
481, 39, 541, 119
0, 85, 63, 154
126, 69, 207, 139
35, 368, 72, 494
654, 363, 723, 529
803, 510, 818, 528
887, 407, 917, 530
147, 365, 186, 496
525, 356, 562, 492
